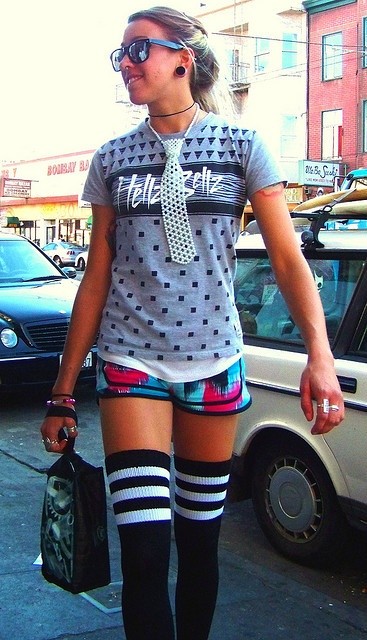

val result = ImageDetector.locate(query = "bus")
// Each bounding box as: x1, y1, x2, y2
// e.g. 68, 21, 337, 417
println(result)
321, 167, 367, 302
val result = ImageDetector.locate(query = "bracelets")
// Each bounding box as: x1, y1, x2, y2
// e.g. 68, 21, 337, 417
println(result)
51, 394, 72, 397
44, 399, 75, 406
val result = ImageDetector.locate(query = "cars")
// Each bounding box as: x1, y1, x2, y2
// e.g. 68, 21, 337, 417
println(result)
40, 240, 83, 267
0, 232, 97, 398
234, 188, 367, 568
74, 244, 89, 270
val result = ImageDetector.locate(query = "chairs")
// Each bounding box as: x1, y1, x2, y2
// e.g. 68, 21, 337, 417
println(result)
310, 260, 343, 346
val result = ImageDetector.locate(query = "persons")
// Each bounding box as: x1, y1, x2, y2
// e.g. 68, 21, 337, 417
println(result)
40, 7, 345, 640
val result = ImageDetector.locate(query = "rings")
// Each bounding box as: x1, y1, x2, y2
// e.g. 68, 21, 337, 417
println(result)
317, 399, 328, 414
44, 436, 56, 451
41, 439, 44, 444
329, 405, 338, 410
66, 425, 77, 432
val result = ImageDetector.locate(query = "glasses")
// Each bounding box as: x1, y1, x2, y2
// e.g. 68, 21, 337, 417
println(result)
110, 38, 184, 72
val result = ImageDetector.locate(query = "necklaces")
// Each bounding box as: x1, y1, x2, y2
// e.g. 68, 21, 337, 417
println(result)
147, 101, 199, 264
148, 102, 195, 117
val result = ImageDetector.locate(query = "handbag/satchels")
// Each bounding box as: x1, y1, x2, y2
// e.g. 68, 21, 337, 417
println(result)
41, 405, 112, 596
